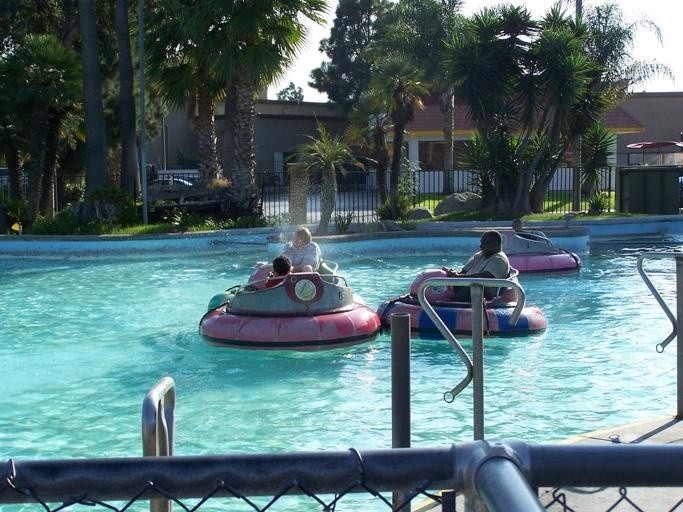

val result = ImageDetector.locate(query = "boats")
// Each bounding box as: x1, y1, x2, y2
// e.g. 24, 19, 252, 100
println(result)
497, 218, 582, 278
197, 258, 383, 352
375, 265, 548, 340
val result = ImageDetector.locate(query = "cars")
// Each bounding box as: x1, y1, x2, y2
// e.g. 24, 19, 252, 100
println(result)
139, 178, 196, 199
0, 166, 29, 191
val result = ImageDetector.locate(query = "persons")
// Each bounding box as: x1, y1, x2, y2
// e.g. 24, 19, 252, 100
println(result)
280, 228, 322, 272
512, 219, 541, 241
442, 230, 510, 302
264, 255, 293, 289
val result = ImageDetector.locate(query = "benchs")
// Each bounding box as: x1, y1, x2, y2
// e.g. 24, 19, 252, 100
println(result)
157, 169, 223, 189
